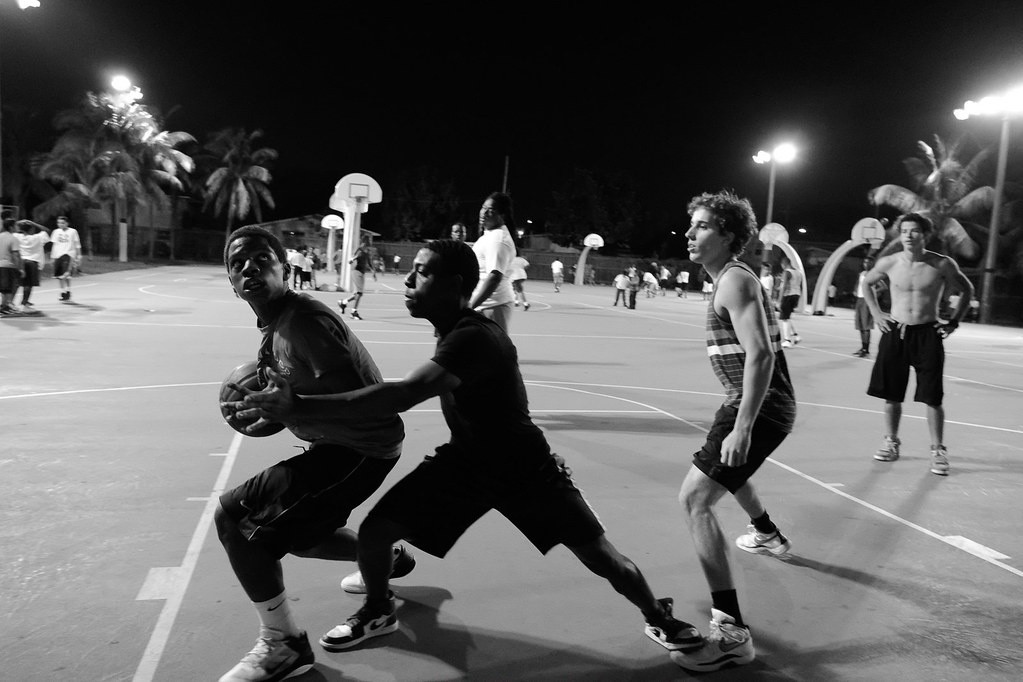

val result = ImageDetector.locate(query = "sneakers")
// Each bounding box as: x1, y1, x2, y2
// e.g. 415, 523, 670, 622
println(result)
872, 435, 902, 461
319, 589, 397, 648
217, 625, 314, 682
671, 611, 755, 672
644, 597, 703, 650
340, 543, 416, 593
930, 444, 951, 474
736, 524, 793, 556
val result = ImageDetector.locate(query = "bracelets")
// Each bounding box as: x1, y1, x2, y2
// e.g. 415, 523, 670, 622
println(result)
949, 320, 959, 328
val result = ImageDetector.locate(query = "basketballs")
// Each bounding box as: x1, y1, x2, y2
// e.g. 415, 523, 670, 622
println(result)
218, 362, 286, 437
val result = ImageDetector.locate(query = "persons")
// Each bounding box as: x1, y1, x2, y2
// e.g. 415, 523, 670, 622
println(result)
214, 226, 416, 682
775, 258, 803, 347
828, 282, 837, 307
286, 245, 321, 290
863, 213, 973, 475
0, 210, 81, 315
852, 256, 887, 356
948, 291, 979, 323
235, 240, 704, 650
675, 271, 689, 298
337, 233, 375, 320
670, 195, 798, 671
759, 263, 774, 296
451, 223, 466, 242
468, 192, 528, 334
393, 253, 401, 272
702, 279, 713, 300
551, 256, 564, 292
612, 262, 671, 309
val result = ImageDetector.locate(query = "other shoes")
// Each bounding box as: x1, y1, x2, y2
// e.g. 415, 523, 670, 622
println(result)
21, 300, 34, 306
59, 291, 71, 301
338, 299, 347, 314
780, 338, 794, 348
350, 310, 362, 321
0, 302, 20, 314
853, 348, 870, 357
790, 334, 800, 342
